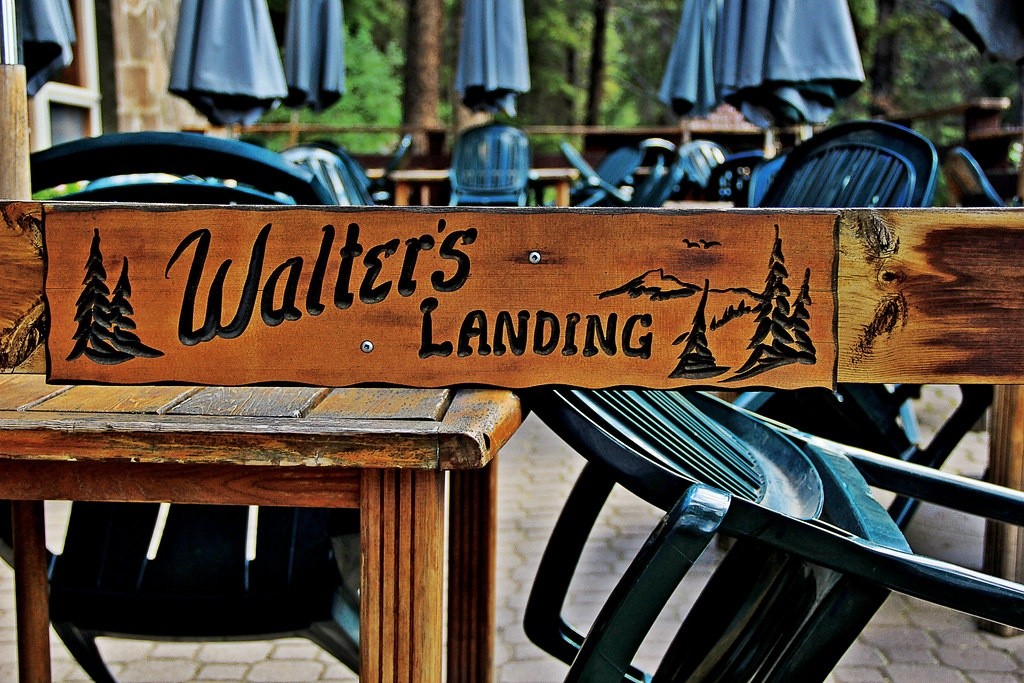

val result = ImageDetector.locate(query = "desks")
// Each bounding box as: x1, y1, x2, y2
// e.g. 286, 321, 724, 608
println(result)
366, 168, 579, 206
575, 128, 801, 169
0, 360, 535, 683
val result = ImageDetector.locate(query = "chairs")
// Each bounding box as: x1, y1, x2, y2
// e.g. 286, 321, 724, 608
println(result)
29, 120, 1024, 683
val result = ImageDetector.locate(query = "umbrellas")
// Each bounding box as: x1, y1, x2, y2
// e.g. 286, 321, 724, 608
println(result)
169, 0, 345, 128
454, 0, 530, 119
659, 0, 869, 132
926, 0, 1024, 72
0, 1, 77, 98
